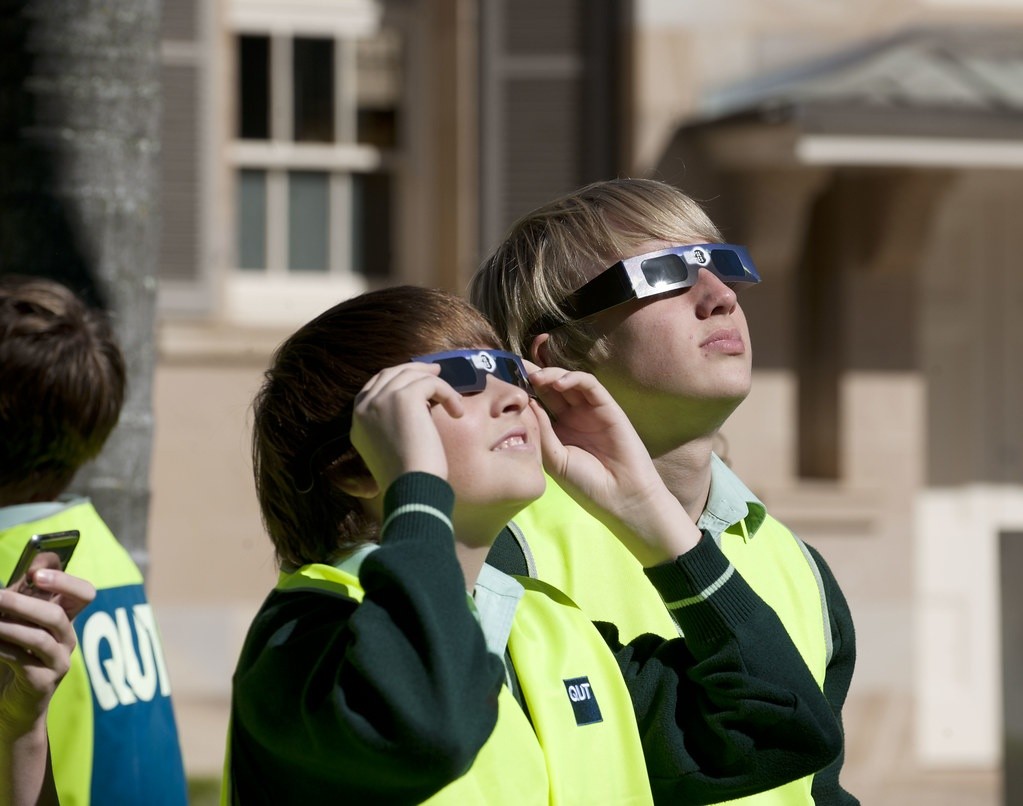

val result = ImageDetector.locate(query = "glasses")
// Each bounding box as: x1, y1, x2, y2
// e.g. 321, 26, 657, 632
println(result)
416, 353, 537, 396
526, 241, 763, 350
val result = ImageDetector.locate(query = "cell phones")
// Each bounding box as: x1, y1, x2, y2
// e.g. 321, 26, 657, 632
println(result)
6, 530, 79, 601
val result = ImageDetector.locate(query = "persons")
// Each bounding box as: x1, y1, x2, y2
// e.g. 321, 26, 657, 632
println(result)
226, 287, 840, 806
1, 278, 194, 806
0, 562, 96, 806
464, 175, 868, 806
